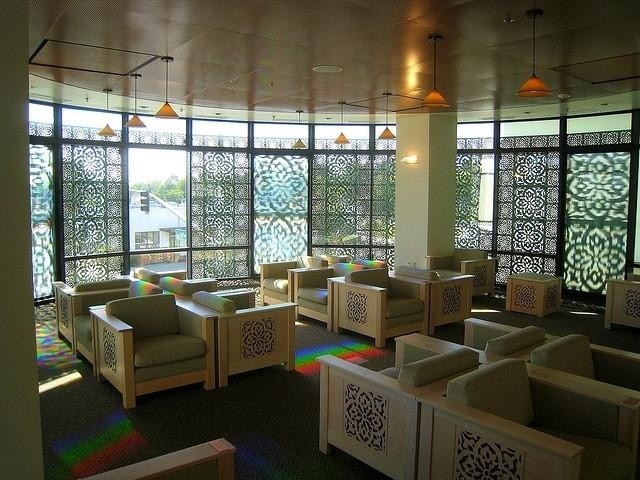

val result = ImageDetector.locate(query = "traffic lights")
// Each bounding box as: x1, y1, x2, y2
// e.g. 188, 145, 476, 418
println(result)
140, 190, 151, 214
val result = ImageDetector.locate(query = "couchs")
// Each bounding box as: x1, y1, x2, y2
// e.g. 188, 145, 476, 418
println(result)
52, 266, 298, 411
260, 248, 498, 352
315, 318, 640, 480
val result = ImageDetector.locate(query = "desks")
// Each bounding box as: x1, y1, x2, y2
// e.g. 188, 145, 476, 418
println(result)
505, 271, 563, 317
603, 278, 639, 330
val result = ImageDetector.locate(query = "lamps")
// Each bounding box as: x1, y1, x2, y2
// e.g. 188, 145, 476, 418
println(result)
293, 110, 306, 148
514, 9, 552, 98
334, 101, 350, 145
378, 92, 396, 139
155, 55, 179, 120
420, 34, 451, 108
124, 74, 148, 128
97, 88, 119, 138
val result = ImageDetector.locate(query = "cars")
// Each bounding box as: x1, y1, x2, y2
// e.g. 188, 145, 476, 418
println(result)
135, 236, 153, 248
130, 200, 186, 208
